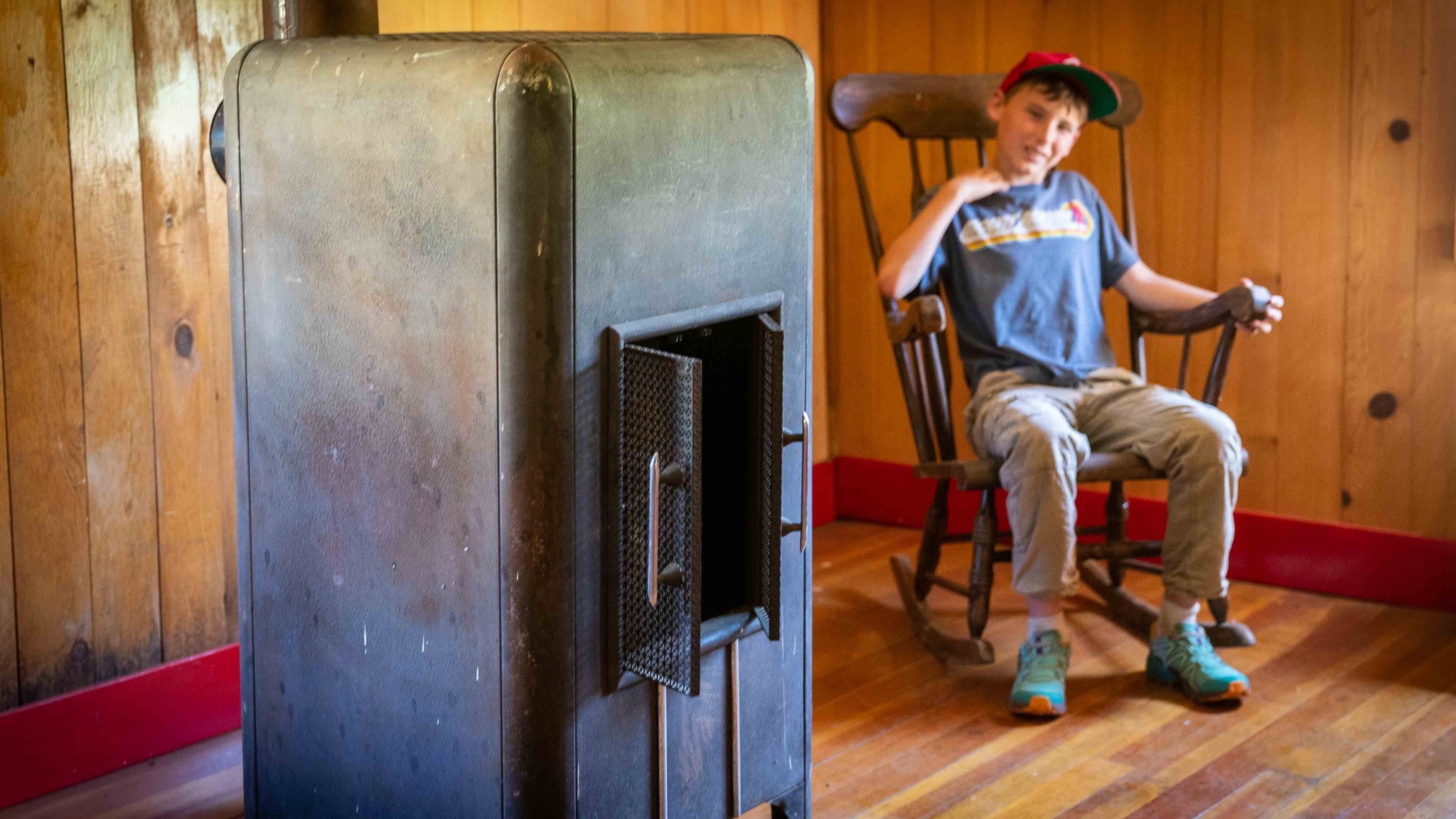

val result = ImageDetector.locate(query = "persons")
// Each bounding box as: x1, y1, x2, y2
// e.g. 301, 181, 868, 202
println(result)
879, 49, 1283, 716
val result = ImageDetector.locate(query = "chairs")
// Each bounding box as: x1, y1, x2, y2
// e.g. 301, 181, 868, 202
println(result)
828, 63, 1258, 662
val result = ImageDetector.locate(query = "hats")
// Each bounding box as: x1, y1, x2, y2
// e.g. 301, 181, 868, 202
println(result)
994, 49, 1123, 122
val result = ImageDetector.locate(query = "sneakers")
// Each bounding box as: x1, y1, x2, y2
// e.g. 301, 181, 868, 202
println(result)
1009, 629, 1073, 716
1144, 621, 1249, 702
